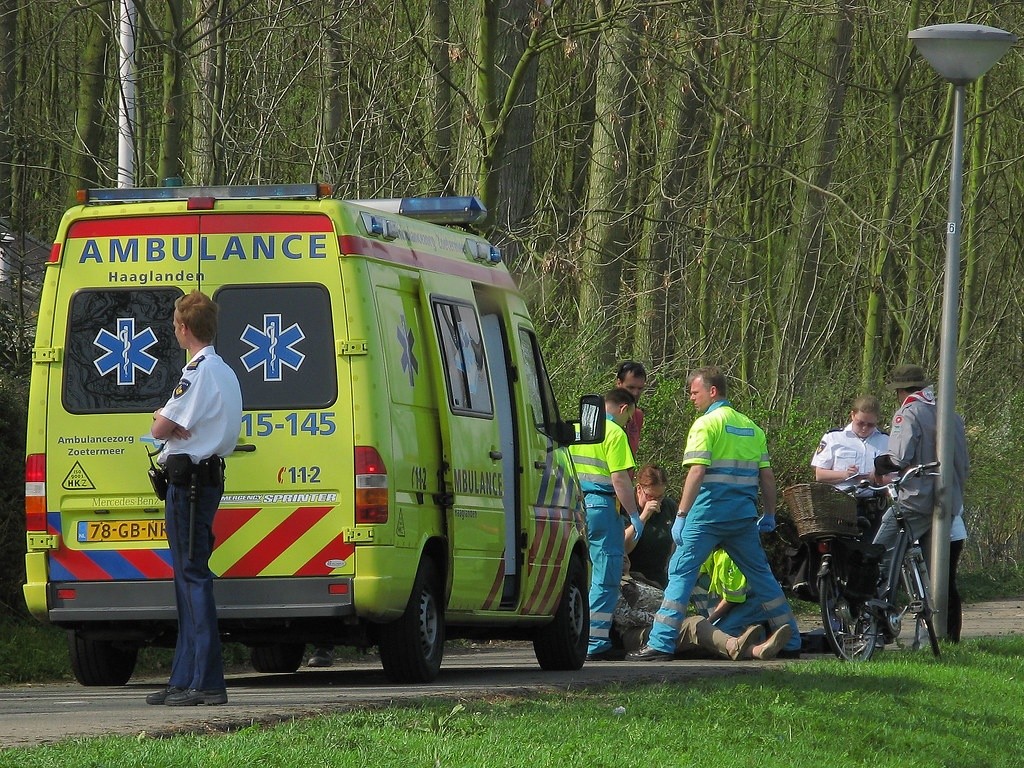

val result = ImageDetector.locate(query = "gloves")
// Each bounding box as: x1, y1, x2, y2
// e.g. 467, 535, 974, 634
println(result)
671, 517, 686, 548
757, 513, 776, 533
630, 514, 644, 543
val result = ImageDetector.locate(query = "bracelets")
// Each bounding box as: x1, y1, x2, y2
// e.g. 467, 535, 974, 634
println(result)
677, 510, 688, 517
153, 417, 156, 420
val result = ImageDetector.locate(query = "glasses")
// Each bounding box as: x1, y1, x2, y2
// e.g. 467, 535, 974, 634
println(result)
641, 485, 666, 500
621, 405, 633, 425
854, 415, 877, 428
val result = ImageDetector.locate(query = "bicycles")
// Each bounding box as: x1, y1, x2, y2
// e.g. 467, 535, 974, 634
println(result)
782, 458, 944, 665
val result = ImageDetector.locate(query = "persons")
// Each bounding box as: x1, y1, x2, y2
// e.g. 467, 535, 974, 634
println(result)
568, 387, 643, 661
613, 554, 792, 661
947, 505, 968, 644
617, 361, 646, 479
811, 394, 901, 619
620, 465, 679, 585
625, 366, 801, 662
845, 365, 971, 651
697, 546, 769, 642
145, 289, 242, 706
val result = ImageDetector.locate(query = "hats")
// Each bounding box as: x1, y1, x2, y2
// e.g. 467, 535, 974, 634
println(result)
886, 364, 928, 390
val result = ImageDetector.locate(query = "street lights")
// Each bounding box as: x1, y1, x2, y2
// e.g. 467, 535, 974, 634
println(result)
902, 20, 1014, 637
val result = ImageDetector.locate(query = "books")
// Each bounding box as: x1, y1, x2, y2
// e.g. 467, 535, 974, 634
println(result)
845, 473, 869, 482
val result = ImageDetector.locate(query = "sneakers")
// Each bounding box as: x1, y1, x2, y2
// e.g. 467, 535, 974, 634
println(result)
165, 684, 229, 707
625, 644, 673, 662
146, 684, 190, 705
308, 647, 334, 667
586, 646, 627, 662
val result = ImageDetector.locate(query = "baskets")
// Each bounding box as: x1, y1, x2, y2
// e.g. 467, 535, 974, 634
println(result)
783, 482, 859, 538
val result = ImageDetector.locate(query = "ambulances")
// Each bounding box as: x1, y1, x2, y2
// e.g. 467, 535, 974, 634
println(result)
21, 179, 608, 688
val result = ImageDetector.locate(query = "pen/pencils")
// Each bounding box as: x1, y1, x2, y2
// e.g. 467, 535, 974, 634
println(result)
844, 459, 860, 473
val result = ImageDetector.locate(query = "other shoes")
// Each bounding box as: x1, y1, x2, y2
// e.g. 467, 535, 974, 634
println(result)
730, 624, 763, 661
758, 623, 791, 661
